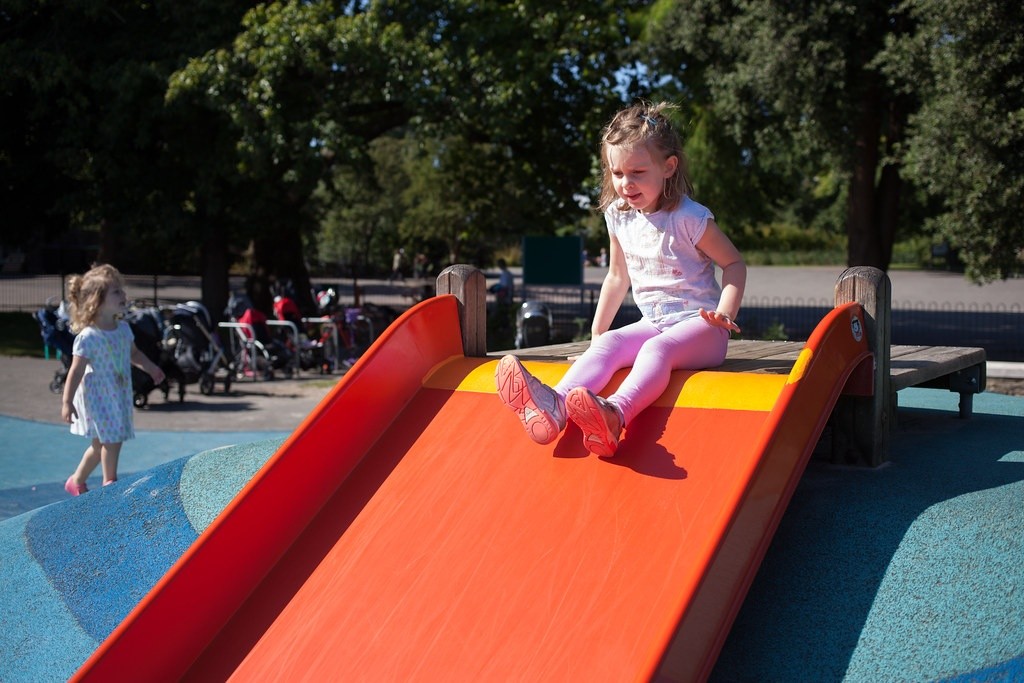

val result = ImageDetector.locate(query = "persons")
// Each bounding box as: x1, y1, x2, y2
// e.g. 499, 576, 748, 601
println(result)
498, 101, 747, 457
62, 263, 165, 496
412, 250, 428, 280
389, 249, 405, 280
492, 258, 514, 338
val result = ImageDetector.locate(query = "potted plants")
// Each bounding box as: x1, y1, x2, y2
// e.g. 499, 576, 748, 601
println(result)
550, 320, 580, 342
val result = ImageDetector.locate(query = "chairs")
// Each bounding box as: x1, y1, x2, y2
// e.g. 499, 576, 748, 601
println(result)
220, 317, 377, 381
520, 300, 553, 347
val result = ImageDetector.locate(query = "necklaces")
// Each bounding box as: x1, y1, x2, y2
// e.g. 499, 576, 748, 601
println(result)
91, 319, 125, 388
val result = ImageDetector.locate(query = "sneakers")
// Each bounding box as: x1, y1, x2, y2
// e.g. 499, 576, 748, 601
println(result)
494, 354, 566, 445
565, 386, 623, 457
65, 476, 88, 496
102, 480, 117, 487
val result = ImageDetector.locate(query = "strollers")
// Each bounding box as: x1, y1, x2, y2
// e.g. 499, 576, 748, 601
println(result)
268, 292, 336, 381
312, 287, 369, 374
513, 300, 554, 352
33, 295, 77, 395
170, 300, 243, 396
221, 292, 297, 383
119, 305, 188, 410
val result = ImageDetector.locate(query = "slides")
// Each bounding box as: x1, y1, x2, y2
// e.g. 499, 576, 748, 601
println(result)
73, 295, 872, 683
